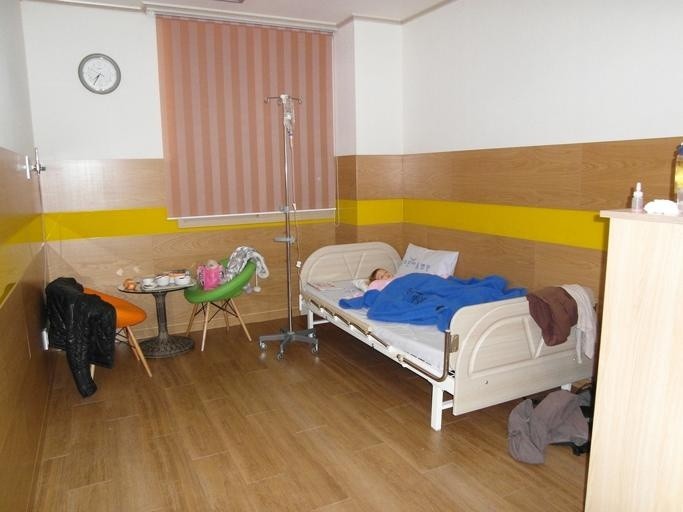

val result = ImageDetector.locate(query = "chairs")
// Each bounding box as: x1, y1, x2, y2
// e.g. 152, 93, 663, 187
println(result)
183, 258, 256, 352
47, 280, 154, 382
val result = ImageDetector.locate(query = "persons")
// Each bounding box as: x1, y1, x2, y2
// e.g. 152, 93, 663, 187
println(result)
369, 269, 395, 292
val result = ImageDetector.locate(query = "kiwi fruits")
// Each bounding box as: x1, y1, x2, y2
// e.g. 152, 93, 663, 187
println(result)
123, 279, 136, 290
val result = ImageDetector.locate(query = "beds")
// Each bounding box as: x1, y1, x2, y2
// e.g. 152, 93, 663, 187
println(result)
298, 241, 596, 431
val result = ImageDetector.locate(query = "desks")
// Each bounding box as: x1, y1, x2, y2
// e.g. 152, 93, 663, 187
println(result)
118, 277, 198, 359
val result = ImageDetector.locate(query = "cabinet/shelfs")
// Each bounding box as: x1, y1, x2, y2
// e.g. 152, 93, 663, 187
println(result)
583, 208, 682, 512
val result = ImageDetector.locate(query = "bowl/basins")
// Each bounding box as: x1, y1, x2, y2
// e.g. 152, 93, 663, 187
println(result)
174, 276, 190, 285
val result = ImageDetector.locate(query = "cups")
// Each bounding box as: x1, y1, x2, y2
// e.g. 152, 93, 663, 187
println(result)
143, 278, 153, 284
676, 189, 682, 216
156, 275, 170, 286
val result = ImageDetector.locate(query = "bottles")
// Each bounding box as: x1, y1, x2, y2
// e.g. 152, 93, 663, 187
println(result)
672, 145, 683, 195
631, 182, 644, 212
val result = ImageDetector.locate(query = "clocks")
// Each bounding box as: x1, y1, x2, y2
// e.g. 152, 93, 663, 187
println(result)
78, 53, 122, 94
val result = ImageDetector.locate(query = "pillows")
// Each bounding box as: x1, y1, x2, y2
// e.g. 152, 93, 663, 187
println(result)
397, 242, 460, 280
350, 276, 372, 292
